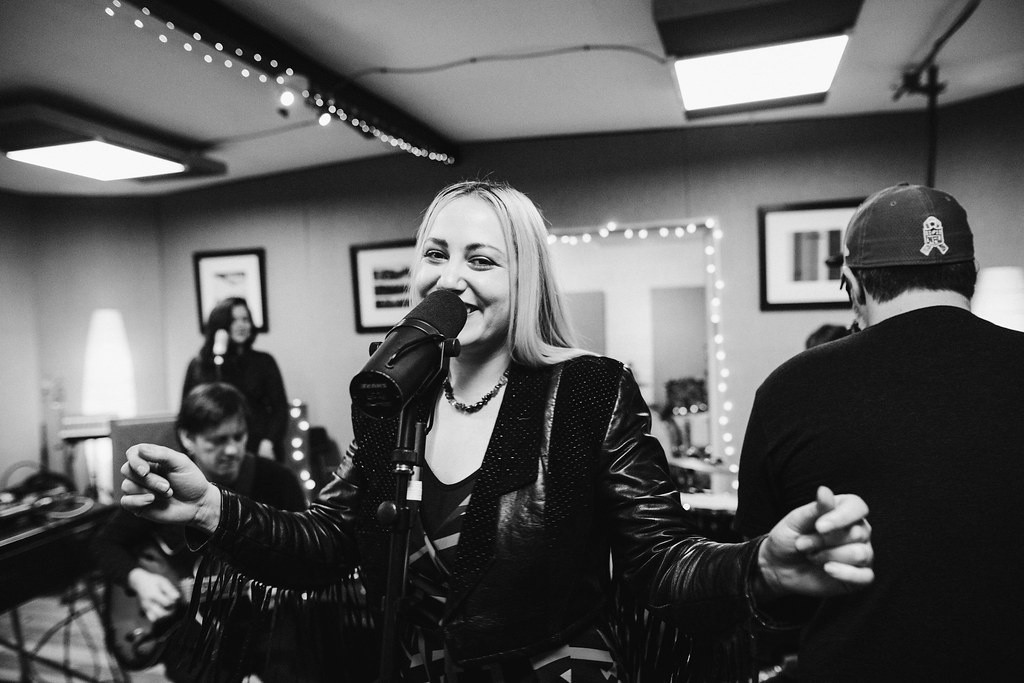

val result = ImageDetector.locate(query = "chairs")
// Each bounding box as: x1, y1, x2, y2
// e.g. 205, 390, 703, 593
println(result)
108, 415, 193, 503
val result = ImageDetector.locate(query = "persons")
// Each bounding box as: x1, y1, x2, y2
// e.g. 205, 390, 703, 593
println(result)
120, 182, 873, 683
182, 298, 290, 462
737, 183, 1024, 683
84, 382, 310, 628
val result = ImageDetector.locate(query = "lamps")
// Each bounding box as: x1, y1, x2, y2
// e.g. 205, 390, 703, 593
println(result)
0, 101, 228, 185
649, 0, 866, 120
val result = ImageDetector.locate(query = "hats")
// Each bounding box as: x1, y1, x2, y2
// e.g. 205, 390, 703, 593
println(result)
840, 181, 975, 289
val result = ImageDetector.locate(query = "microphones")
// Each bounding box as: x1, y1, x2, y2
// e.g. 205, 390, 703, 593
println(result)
212, 328, 230, 365
348, 292, 469, 530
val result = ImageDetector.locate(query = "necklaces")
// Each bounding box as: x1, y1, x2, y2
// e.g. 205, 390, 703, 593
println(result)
443, 369, 509, 412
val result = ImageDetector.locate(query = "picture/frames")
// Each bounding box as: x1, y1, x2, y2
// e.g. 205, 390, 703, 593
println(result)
758, 196, 866, 313
193, 248, 269, 335
349, 238, 422, 334
